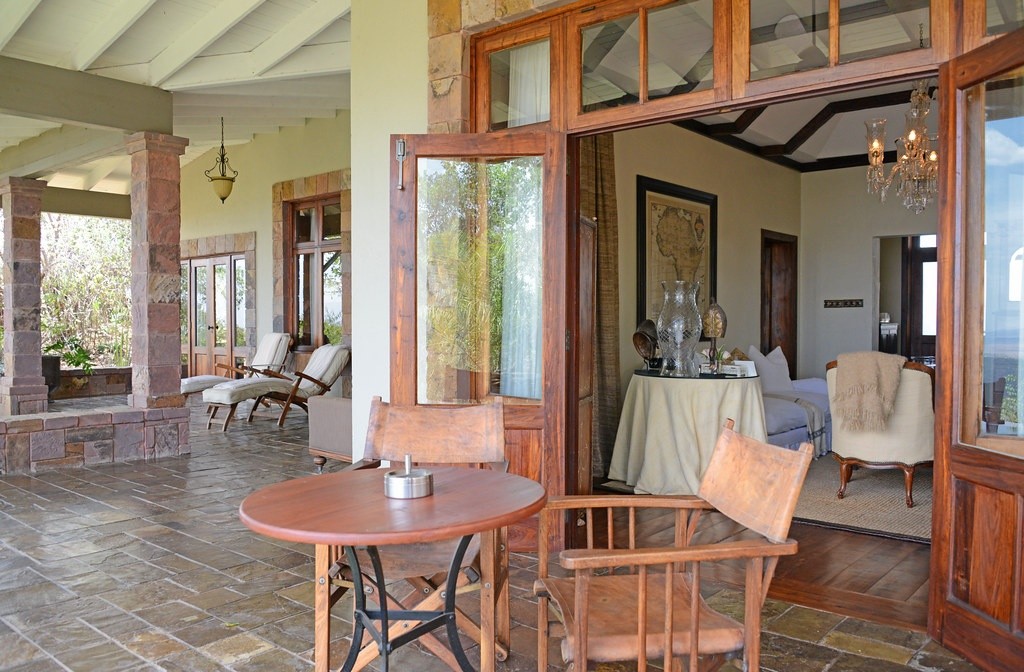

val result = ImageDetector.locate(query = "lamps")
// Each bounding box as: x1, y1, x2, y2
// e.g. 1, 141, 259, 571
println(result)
702, 297, 727, 373
863, 24, 939, 213
205, 117, 238, 205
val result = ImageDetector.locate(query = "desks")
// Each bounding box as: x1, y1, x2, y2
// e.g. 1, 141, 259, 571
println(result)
608, 370, 768, 496
237, 467, 548, 672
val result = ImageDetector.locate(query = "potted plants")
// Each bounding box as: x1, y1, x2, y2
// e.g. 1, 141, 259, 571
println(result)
40, 337, 97, 403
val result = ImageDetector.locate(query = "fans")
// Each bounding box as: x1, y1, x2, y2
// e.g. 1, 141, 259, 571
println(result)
751, 1, 829, 80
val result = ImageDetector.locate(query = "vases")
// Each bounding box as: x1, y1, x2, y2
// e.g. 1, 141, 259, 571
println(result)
655, 281, 703, 378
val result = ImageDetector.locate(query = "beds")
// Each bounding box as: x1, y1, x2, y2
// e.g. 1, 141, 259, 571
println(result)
765, 378, 832, 461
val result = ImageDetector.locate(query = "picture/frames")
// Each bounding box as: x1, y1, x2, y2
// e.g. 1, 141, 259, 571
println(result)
635, 174, 717, 344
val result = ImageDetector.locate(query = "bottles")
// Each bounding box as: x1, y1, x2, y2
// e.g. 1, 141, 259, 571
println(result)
655, 278, 704, 379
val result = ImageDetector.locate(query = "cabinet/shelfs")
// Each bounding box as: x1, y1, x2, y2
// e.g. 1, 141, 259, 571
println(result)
879, 322, 899, 355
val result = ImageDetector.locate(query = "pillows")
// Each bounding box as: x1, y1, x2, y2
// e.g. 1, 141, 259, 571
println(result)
749, 346, 794, 394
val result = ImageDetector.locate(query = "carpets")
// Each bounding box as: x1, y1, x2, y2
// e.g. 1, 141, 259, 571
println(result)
593, 451, 932, 546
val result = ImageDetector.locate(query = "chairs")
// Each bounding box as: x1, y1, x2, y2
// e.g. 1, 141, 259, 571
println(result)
826, 358, 936, 507
181, 333, 294, 414
523, 416, 816, 672
201, 344, 349, 431
311, 396, 511, 672
